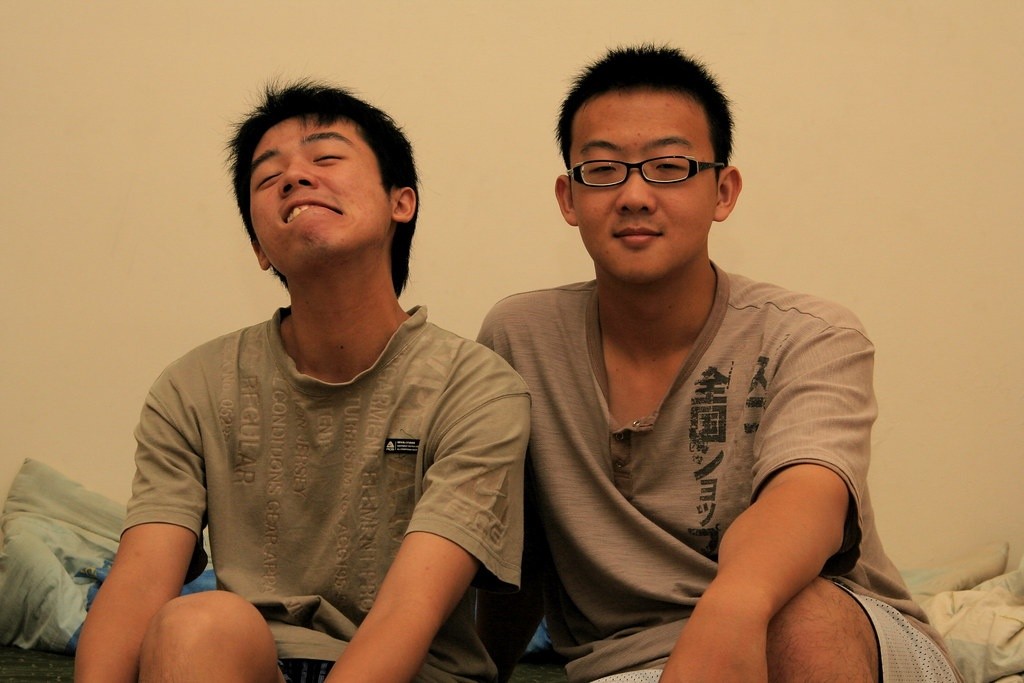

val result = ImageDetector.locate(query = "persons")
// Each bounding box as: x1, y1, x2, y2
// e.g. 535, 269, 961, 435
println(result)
74, 77, 532, 683
475, 45, 967, 683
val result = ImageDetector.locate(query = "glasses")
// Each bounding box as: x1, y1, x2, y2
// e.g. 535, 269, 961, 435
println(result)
568, 155, 725, 187
